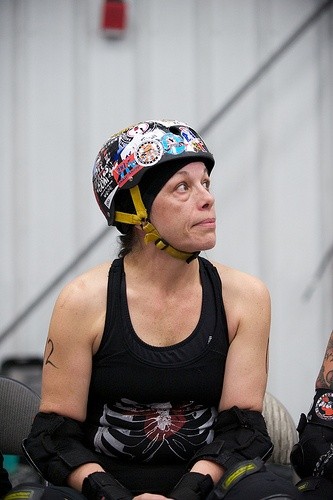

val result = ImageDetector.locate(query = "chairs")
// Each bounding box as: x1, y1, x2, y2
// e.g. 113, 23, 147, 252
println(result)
0, 376, 50, 488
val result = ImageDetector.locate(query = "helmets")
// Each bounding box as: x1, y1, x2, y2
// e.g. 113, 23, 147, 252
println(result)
92, 119, 215, 235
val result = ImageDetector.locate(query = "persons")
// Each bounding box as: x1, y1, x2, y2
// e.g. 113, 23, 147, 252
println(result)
1, 120, 301, 500
295, 331, 333, 500
